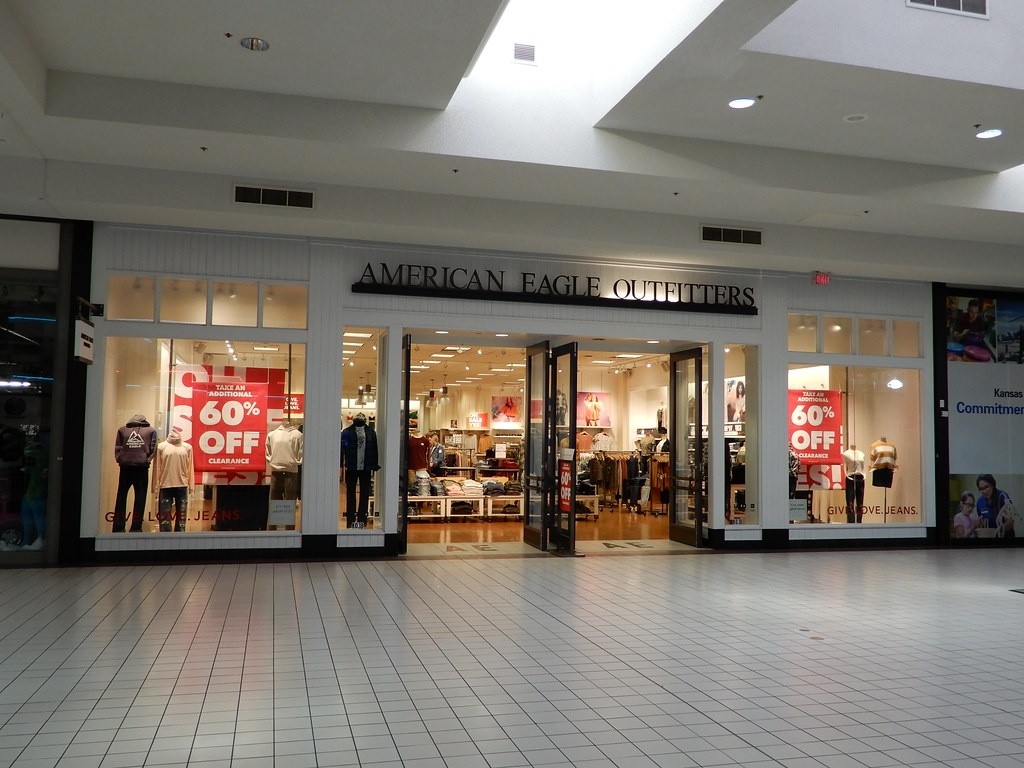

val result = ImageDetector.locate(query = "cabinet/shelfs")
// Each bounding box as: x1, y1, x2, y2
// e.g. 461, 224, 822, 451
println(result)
528, 496, 598, 523
447, 496, 483, 522
368, 496, 446, 522
637, 422, 746, 508
213, 485, 269, 530
494, 429, 524, 459
486, 496, 524, 522
430, 429, 492, 462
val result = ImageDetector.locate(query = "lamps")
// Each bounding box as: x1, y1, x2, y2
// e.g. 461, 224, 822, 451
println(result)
355, 377, 366, 405
363, 372, 374, 403
426, 380, 436, 408
440, 373, 449, 404
662, 361, 670, 371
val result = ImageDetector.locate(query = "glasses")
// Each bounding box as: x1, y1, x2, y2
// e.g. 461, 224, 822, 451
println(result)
962, 502, 977, 508
979, 484, 992, 491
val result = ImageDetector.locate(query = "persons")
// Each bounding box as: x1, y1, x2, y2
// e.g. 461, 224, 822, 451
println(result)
950, 299, 986, 343
556, 390, 568, 426
408, 430, 431, 516
583, 392, 594, 426
150, 432, 196, 532
431, 435, 445, 477
869, 436, 898, 487
339, 416, 381, 528
980, 302, 996, 345
789, 441, 800, 498
976, 474, 1016, 538
640, 432, 656, 477
656, 402, 667, 429
842, 444, 865, 522
264, 418, 305, 532
493, 397, 518, 422
112, 414, 158, 534
427, 435, 436, 475
655, 427, 669, 454
953, 491, 983, 538
589, 394, 601, 426
733, 381, 745, 434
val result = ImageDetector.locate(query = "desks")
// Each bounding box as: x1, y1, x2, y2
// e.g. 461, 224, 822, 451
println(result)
481, 468, 519, 480
440, 467, 475, 481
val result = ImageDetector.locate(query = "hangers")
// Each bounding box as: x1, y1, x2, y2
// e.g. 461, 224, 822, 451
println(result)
648, 454, 670, 462
433, 430, 490, 437
580, 431, 589, 435
601, 432, 611, 437
594, 451, 635, 461
